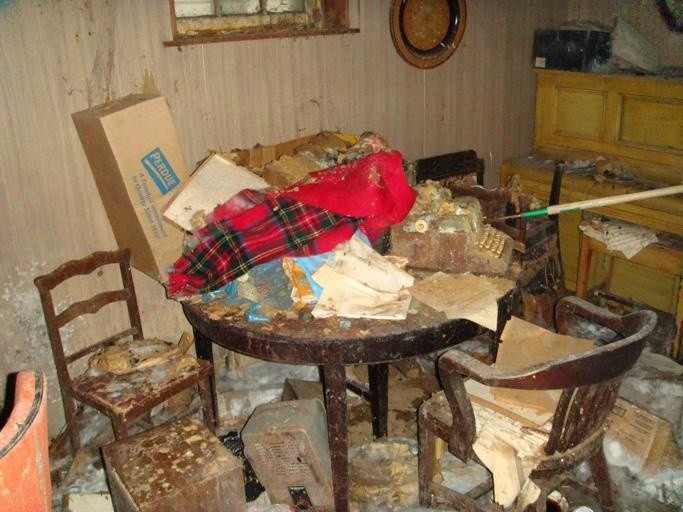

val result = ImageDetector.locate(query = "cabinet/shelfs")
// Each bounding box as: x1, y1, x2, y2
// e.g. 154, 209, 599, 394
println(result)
529, 67, 682, 184
499, 154, 681, 317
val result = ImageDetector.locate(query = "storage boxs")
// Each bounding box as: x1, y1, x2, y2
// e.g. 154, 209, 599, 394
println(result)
69, 92, 191, 284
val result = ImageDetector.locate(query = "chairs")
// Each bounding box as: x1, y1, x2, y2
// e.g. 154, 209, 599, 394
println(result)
34, 248, 214, 450
416, 297, 658, 511
456, 185, 564, 293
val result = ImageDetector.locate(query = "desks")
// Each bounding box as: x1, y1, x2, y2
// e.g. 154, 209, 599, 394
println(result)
182, 286, 515, 511
